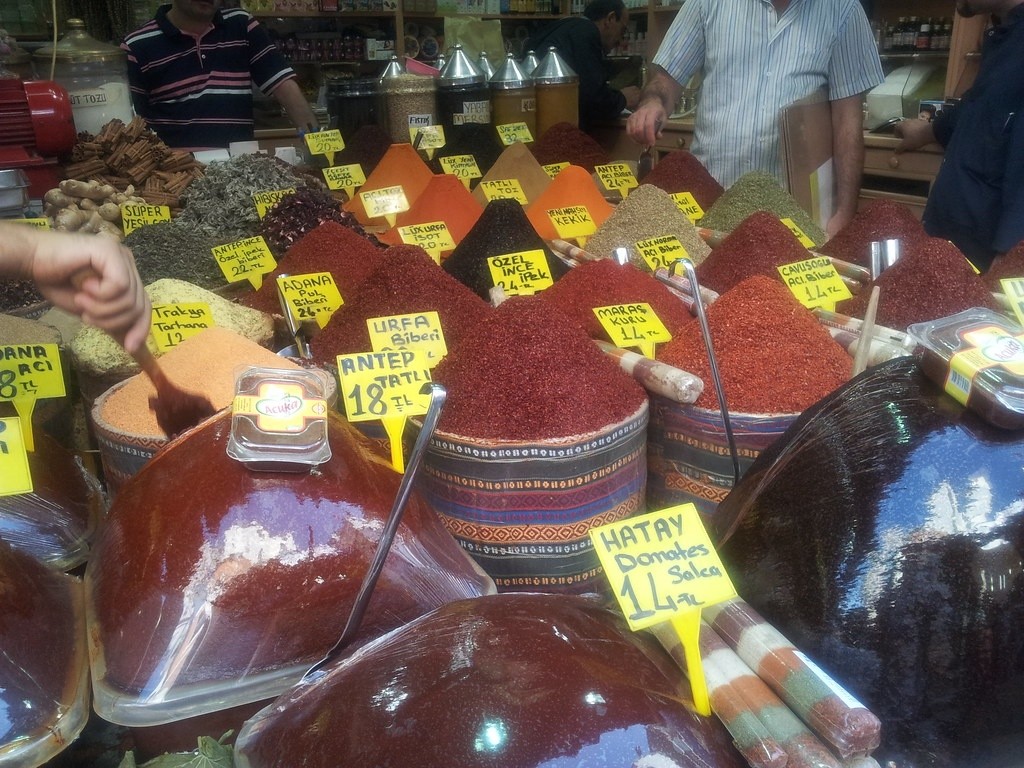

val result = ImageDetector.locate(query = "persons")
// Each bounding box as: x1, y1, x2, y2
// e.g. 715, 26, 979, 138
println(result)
627, 0, 886, 238
0, 220, 152, 352
890, 0, 1024, 275
121, 0, 319, 147
523, 0, 640, 126
920, 104, 936, 122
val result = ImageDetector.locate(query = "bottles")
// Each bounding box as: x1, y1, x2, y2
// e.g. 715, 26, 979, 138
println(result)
873, 13, 951, 53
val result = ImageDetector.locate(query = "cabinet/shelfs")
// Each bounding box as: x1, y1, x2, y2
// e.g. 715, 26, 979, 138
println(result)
228, 1, 991, 221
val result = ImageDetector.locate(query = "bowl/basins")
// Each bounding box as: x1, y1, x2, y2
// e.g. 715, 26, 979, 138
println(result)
226, 363, 338, 475
906, 306, 1024, 433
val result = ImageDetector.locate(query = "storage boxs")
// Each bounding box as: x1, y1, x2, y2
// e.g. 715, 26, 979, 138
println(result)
225, 362, 338, 468
905, 305, 1023, 430
